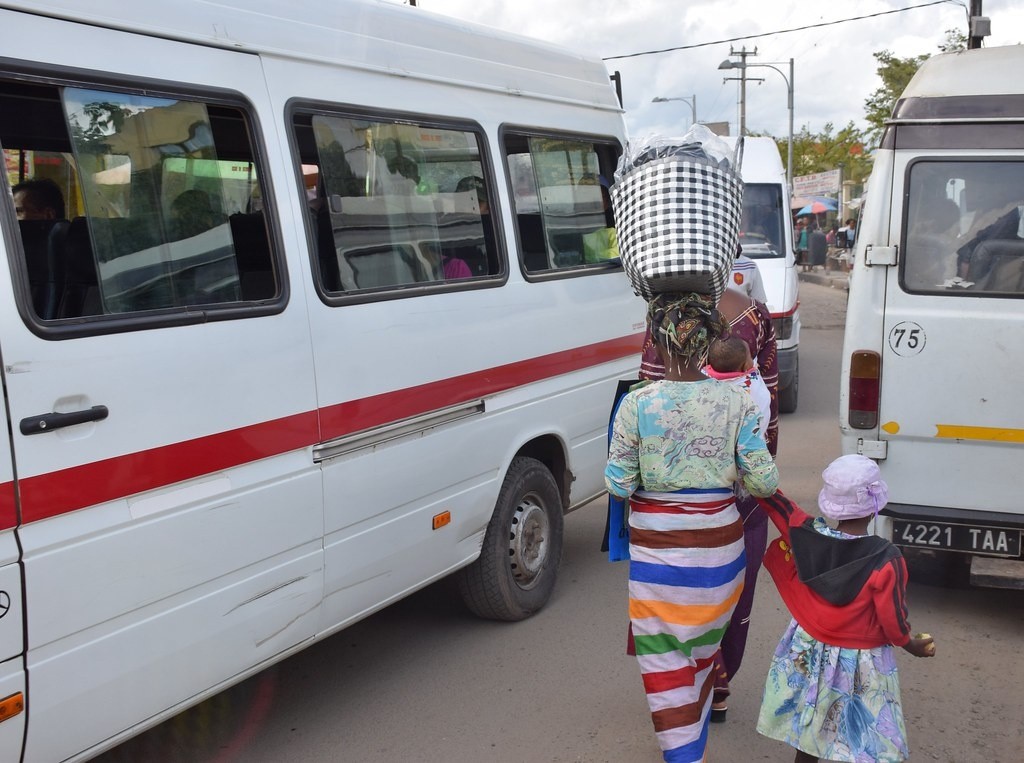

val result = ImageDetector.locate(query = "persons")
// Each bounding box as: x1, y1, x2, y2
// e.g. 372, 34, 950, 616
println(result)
754, 455, 936, 763
914, 194, 1021, 280
604, 254, 778, 763
794, 217, 856, 248
740, 208, 766, 240
10, 173, 620, 319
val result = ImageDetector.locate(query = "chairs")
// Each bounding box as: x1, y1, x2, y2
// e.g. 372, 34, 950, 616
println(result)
18, 211, 554, 326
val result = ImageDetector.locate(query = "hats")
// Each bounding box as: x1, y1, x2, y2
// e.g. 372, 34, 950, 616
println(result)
817, 454, 889, 523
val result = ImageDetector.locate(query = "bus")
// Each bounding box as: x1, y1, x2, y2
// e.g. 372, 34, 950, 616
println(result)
0, 0, 648, 763
716, 133, 830, 415
838, 41, 1024, 590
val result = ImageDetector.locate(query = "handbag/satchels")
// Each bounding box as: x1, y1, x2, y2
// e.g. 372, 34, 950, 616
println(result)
608, 133, 744, 304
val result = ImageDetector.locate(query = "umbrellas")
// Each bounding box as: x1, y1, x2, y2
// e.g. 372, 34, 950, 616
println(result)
795, 201, 838, 229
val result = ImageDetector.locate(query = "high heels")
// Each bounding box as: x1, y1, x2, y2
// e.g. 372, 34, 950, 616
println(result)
710, 707, 728, 723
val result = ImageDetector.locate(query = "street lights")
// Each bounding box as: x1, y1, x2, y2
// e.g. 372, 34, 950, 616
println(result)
651, 93, 697, 124
716, 57, 795, 207
